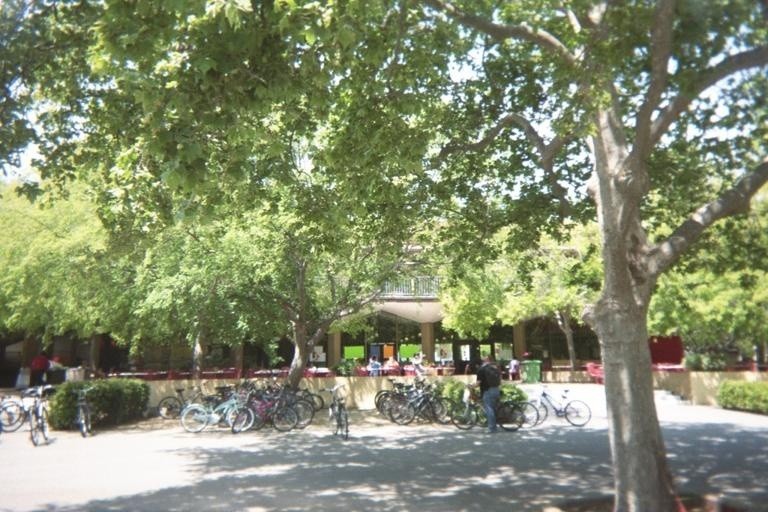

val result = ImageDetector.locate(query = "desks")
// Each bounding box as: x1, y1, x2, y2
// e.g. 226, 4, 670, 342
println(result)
107, 364, 457, 381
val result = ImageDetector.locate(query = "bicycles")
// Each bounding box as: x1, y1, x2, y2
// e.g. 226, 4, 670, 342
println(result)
0, 385, 56, 446
373, 376, 538, 433
181, 378, 324, 434
319, 384, 351, 441
70, 385, 98, 438
530, 385, 591, 427
157, 382, 208, 420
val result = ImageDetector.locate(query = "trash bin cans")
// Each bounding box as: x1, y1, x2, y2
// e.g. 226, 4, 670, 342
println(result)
519, 359, 543, 381
65, 368, 84, 381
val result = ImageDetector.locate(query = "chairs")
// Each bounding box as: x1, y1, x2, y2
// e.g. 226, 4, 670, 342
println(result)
511, 364, 519, 380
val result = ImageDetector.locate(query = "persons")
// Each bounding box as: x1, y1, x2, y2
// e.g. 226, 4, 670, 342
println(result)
466, 358, 504, 432
368, 351, 436, 376
508, 356, 521, 380
29, 351, 83, 387
